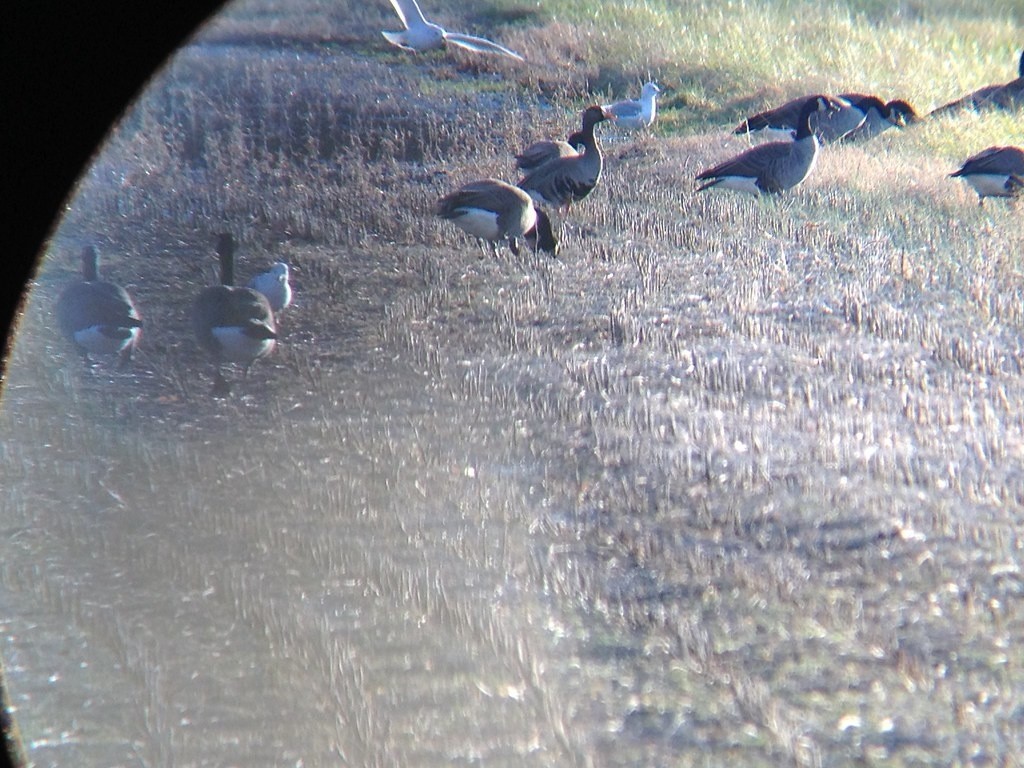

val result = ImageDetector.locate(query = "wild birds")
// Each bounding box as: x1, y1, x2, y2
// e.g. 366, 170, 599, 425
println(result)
56, 244, 144, 367
512, 132, 600, 177
730, 95, 907, 148
923, 50, 1024, 118
838, 92, 918, 146
193, 232, 277, 398
947, 145, 1024, 208
247, 263, 291, 313
517, 106, 617, 221
435, 178, 561, 258
381, 0, 524, 63
601, 83, 661, 130
694, 94, 840, 198
